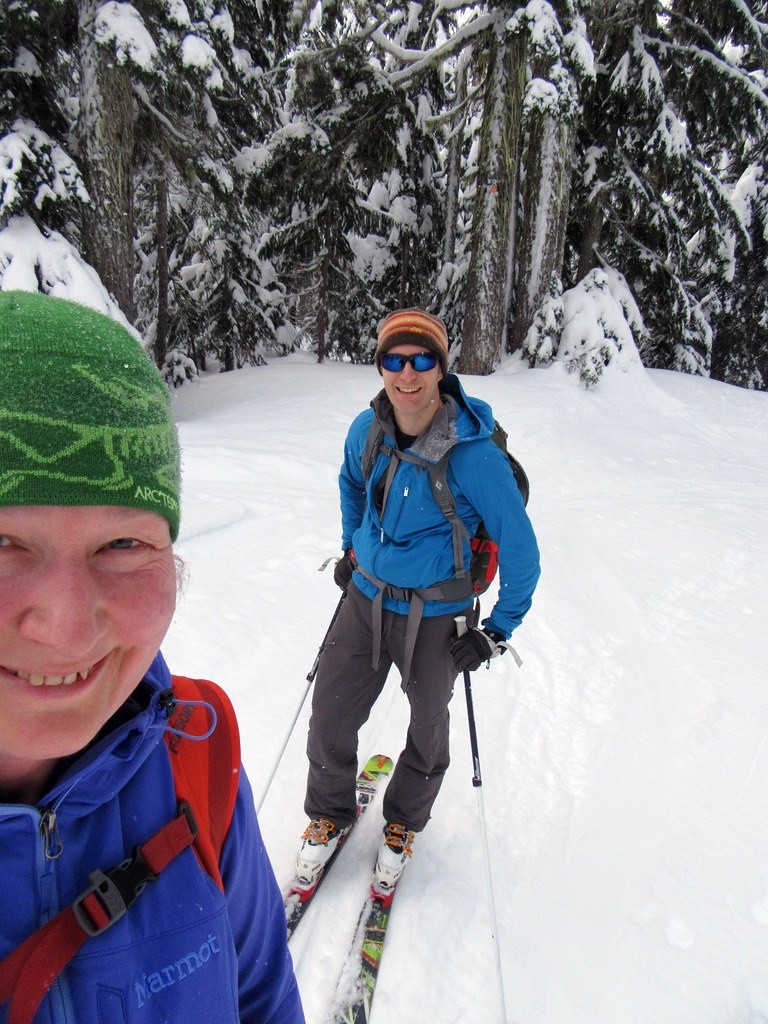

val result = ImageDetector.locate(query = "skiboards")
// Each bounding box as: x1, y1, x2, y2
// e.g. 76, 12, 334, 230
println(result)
283, 752, 406, 1024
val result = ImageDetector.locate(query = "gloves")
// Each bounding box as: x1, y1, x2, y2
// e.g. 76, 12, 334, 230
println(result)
449, 627, 513, 674
333, 550, 354, 590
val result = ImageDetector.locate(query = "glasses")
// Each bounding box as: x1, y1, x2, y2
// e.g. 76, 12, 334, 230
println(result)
379, 352, 440, 373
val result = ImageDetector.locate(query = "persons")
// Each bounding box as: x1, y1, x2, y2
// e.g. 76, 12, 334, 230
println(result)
287, 308, 542, 909
1, 289, 307, 1024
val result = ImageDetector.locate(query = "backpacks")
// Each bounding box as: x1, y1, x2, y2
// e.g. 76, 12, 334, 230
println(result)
427, 414, 528, 597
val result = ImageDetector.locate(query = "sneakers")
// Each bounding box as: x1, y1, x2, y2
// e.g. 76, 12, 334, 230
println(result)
296, 817, 344, 880
377, 822, 416, 888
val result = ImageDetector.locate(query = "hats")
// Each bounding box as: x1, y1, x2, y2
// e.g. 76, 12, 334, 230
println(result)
375, 308, 448, 380
0, 290, 179, 544
